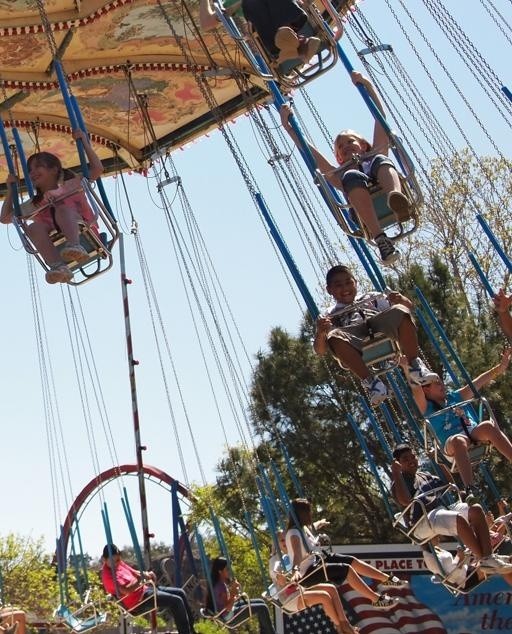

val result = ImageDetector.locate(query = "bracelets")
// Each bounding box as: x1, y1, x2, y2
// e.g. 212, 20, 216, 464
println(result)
489, 368, 500, 378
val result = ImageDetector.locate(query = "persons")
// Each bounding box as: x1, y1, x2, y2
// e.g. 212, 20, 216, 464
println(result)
101, 542, 200, 634
314, 265, 439, 405
269, 525, 361, 634
0, 126, 103, 284
241, 0, 321, 65
285, 498, 411, 609
420, 497, 512, 592
203, 555, 276, 634
397, 343, 512, 505
492, 287, 512, 347
391, 443, 512, 575
279, 69, 411, 266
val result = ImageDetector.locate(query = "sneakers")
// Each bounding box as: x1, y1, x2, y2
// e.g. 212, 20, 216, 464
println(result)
480, 553, 512, 574
375, 233, 401, 264
275, 27, 320, 63
363, 375, 387, 406
388, 191, 411, 222
372, 595, 399, 608
408, 357, 438, 387
46, 264, 74, 284
466, 486, 482, 505
61, 246, 89, 261
380, 575, 408, 588
